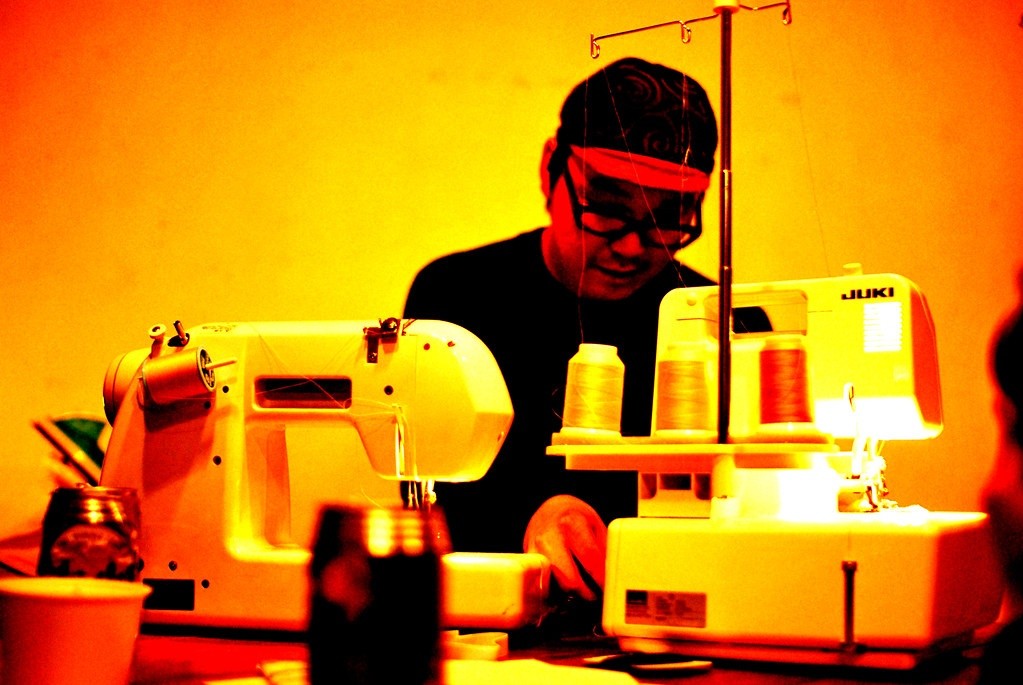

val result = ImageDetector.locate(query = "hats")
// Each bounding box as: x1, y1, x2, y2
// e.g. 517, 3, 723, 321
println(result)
556, 57, 719, 194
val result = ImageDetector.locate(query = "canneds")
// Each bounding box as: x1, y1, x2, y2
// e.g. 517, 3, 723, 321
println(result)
35, 485, 147, 586
301, 504, 453, 685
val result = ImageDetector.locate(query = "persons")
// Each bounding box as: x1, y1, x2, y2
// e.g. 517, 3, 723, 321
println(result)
906, 306, 1023, 685
396, 55, 772, 653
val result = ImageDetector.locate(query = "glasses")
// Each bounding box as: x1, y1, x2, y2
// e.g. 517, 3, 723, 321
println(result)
557, 127, 702, 252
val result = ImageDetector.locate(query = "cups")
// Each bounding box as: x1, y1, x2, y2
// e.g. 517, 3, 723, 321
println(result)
0, 576, 152, 685
